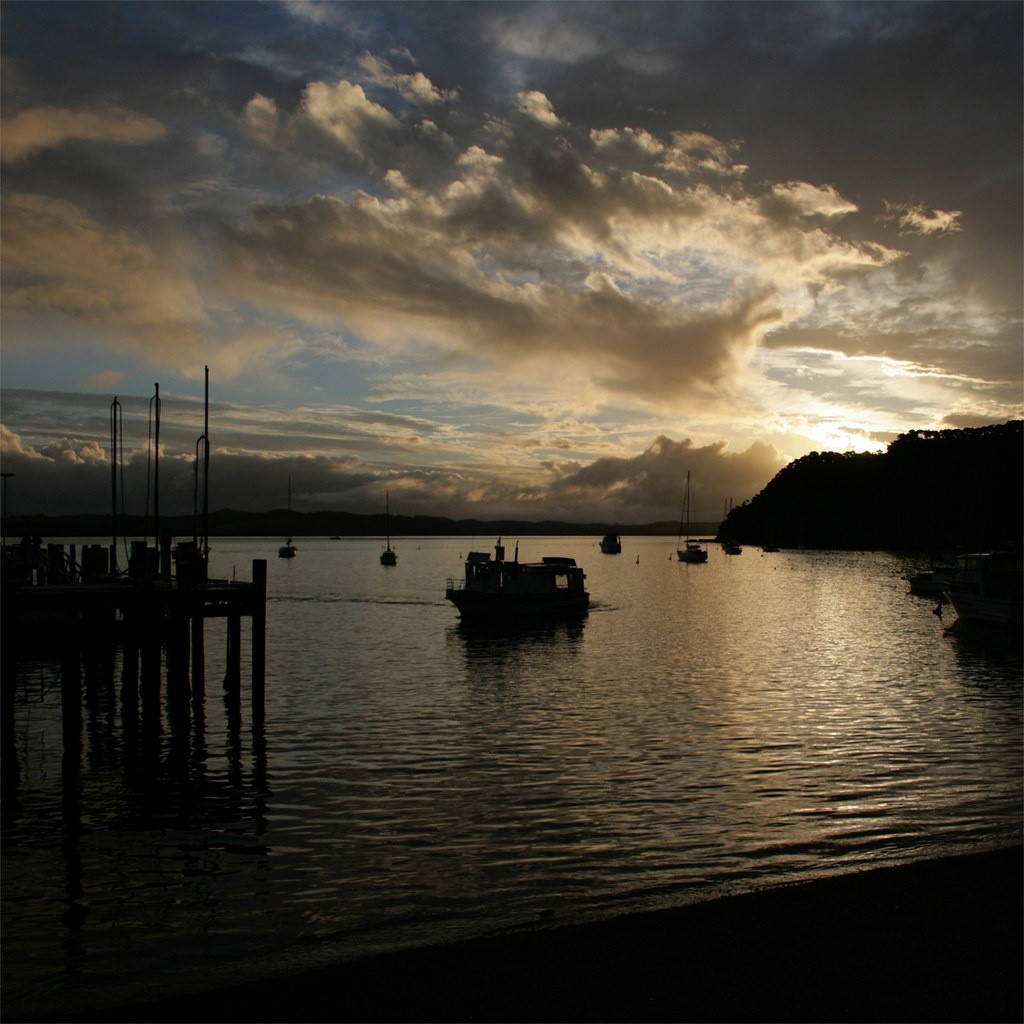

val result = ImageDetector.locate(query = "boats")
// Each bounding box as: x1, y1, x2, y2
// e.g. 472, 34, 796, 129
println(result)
599, 532, 622, 555
905, 550, 1024, 625
444, 535, 591, 625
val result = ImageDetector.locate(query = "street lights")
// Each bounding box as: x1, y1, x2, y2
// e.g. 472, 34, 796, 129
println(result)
0, 473, 15, 552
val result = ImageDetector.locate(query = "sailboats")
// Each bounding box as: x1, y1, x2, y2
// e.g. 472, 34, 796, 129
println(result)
380, 491, 397, 565
677, 470, 708, 561
762, 500, 779, 552
278, 475, 297, 557
721, 497, 742, 554
